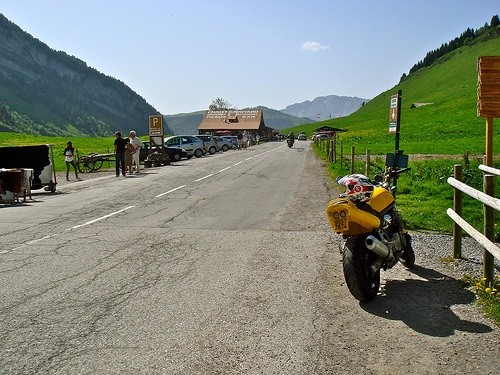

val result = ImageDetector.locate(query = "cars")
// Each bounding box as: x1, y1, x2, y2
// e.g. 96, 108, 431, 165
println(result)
133, 141, 188, 164
146, 135, 206, 159
221, 136, 239, 150
297, 134, 306, 141
194, 135, 218, 155
275, 133, 297, 140
208, 136, 231, 152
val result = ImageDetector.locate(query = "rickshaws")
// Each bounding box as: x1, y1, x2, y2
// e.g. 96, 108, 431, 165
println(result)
76, 153, 116, 174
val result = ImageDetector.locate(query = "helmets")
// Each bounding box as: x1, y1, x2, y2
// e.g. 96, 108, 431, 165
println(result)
337, 174, 375, 203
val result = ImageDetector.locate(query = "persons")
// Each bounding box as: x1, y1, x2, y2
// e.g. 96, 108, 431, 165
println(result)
256, 133, 260, 145
124, 137, 134, 175
247, 131, 252, 146
114, 131, 128, 177
129, 131, 143, 174
63, 141, 81, 181
275, 131, 294, 146
237, 131, 243, 150
242, 130, 248, 150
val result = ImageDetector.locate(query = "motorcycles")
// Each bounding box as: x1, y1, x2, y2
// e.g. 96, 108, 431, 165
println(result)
326, 167, 415, 301
287, 136, 294, 149
